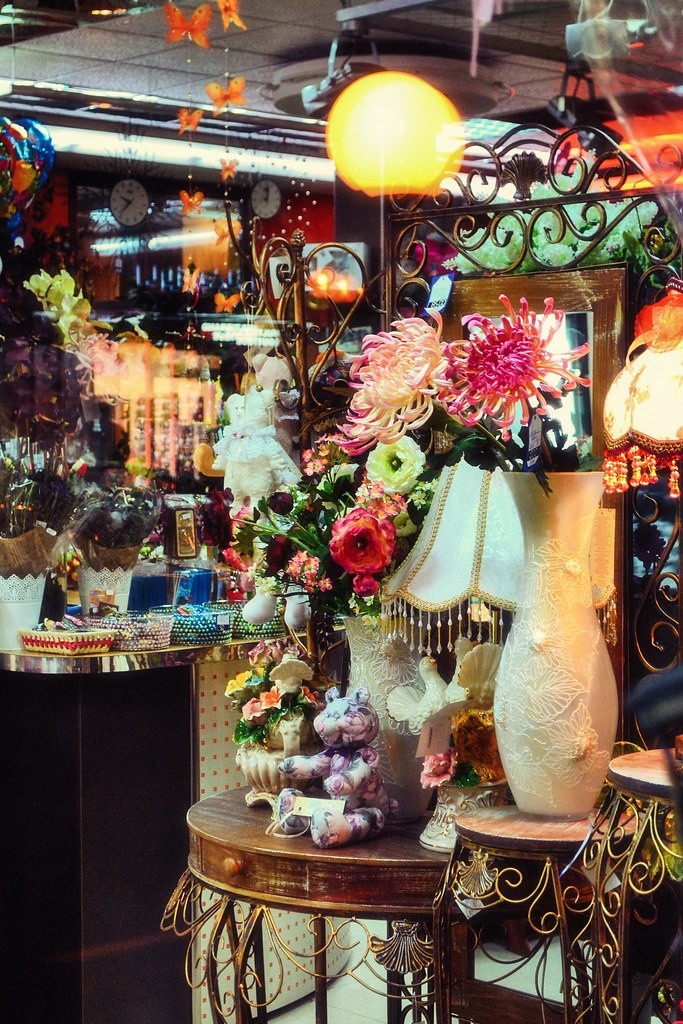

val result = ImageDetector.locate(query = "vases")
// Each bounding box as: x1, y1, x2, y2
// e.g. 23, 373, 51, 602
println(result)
335, 615, 435, 825
491, 472, 617, 825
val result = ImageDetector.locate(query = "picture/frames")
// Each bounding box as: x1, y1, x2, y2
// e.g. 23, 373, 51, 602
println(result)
429, 267, 628, 731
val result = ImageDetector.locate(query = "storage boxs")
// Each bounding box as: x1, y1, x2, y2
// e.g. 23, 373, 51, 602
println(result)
150, 604, 236, 646
211, 602, 282, 637
84, 615, 172, 650
20, 628, 117, 655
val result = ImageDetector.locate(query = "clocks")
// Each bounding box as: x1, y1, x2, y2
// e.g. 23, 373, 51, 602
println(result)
251, 177, 283, 219
109, 181, 150, 225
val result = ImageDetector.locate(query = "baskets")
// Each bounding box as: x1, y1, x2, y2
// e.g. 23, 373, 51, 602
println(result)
20, 600, 285, 656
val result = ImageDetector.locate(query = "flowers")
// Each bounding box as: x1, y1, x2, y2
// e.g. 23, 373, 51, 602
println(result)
419, 745, 484, 790
227, 435, 439, 638
333, 294, 587, 499
224, 636, 316, 745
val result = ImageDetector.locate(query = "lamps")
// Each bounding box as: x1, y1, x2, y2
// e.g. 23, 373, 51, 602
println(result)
379, 453, 617, 653
549, 97, 624, 157
303, 57, 385, 120
603, 274, 683, 498
324, 70, 466, 193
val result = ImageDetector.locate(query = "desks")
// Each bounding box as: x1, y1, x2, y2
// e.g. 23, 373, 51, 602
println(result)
159, 784, 619, 1024
557, 749, 683, 1024
431, 804, 647, 1024
